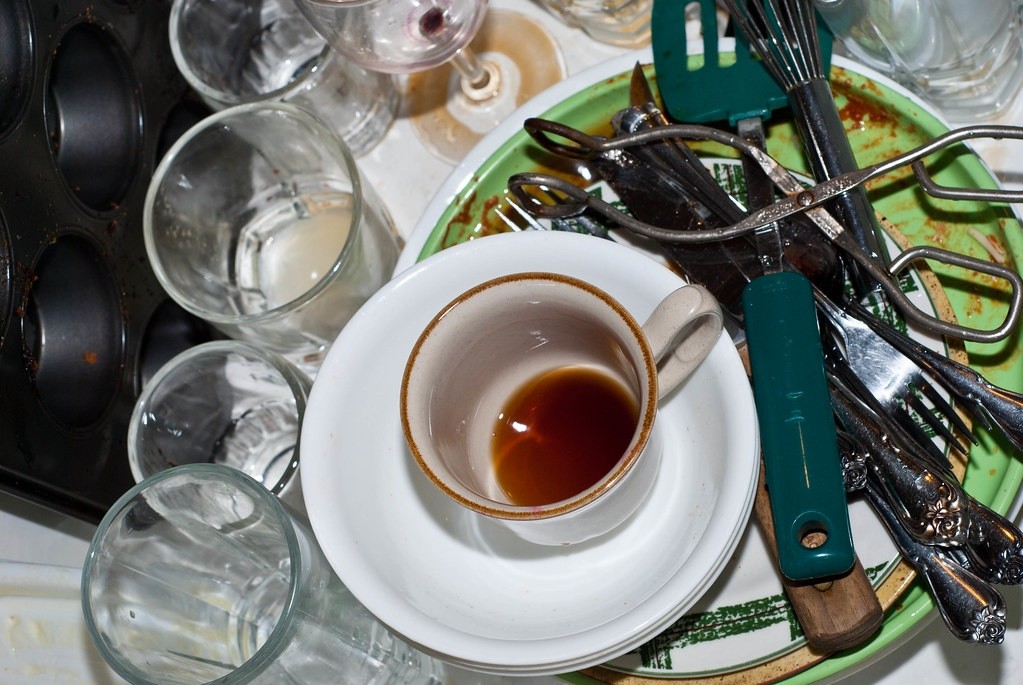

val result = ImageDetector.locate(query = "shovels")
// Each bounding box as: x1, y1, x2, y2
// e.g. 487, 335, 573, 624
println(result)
652, 0, 857, 580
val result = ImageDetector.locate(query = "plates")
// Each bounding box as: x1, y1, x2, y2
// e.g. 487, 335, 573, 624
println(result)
300, 230, 761, 677
524, 158, 955, 678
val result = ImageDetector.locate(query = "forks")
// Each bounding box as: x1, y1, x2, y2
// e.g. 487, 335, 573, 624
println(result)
469, 196, 547, 241
607, 107, 992, 469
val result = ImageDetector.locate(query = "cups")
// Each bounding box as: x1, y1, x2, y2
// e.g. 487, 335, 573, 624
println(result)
815, 0, 1023, 121
168, 1, 397, 156
143, 103, 405, 370
399, 273, 723, 547
128, 339, 310, 532
539, 0, 652, 48
81, 463, 443, 684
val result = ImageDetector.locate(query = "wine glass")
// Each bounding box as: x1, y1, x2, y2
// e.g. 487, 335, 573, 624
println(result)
298, 1, 568, 167
